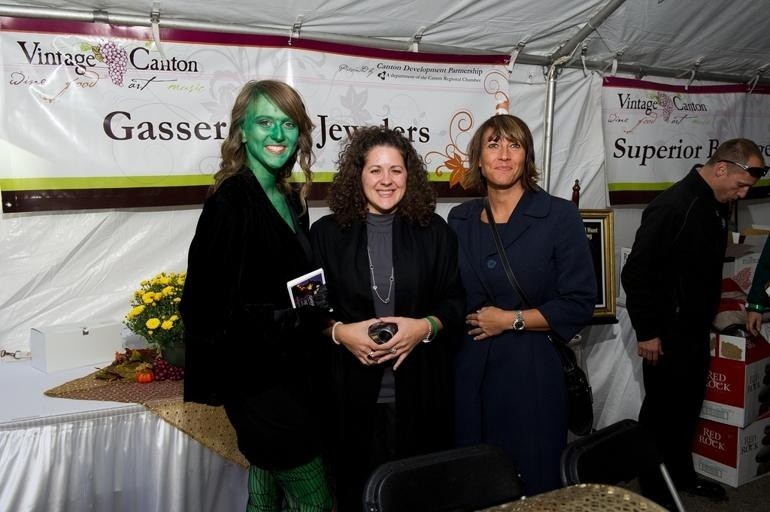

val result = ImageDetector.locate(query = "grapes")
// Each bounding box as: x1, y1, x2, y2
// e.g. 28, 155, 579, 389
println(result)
153, 357, 184, 381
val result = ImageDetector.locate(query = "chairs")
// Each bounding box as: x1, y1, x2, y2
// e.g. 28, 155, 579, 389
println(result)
561, 418, 687, 511
361, 436, 521, 512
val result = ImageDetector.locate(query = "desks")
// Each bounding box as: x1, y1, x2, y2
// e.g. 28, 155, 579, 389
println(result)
471, 481, 670, 511
1, 348, 254, 512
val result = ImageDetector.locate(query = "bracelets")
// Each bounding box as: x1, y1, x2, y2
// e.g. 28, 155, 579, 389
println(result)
745, 303, 765, 312
427, 316, 438, 341
421, 318, 431, 344
331, 320, 343, 346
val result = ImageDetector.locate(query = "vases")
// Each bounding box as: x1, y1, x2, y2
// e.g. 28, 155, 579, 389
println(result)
159, 341, 186, 369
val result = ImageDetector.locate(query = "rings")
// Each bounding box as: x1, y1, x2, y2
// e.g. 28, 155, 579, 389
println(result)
390, 345, 398, 353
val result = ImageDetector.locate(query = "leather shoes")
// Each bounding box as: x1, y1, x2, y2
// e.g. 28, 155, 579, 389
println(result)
683, 476, 726, 501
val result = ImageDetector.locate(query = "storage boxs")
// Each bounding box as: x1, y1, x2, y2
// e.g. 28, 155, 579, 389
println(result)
702, 336, 770, 427
689, 417, 770, 491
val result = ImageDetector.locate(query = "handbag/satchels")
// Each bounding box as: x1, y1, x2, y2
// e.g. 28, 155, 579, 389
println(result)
562, 343, 595, 438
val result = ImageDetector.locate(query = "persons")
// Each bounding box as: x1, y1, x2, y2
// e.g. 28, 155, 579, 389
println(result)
745, 228, 770, 337
447, 114, 598, 512
619, 137, 769, 512
176, 79, 329, 511
308, 125, 464, 511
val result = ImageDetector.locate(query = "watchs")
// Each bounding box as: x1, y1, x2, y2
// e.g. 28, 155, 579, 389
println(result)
513, 309, 525, 332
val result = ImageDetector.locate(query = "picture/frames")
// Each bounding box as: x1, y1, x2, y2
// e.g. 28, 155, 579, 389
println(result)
578, 208, 616, 319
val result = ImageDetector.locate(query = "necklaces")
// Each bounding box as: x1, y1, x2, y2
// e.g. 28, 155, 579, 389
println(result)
366, 234, 394, 305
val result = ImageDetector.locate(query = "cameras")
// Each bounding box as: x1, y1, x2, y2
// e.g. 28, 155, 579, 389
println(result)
369, 323, 397, 344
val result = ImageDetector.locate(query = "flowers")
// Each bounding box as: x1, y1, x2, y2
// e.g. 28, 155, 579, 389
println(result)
121, 269, 189, 350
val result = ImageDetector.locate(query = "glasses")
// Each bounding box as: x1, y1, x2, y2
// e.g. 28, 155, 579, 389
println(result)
730, 160, 769, 178
